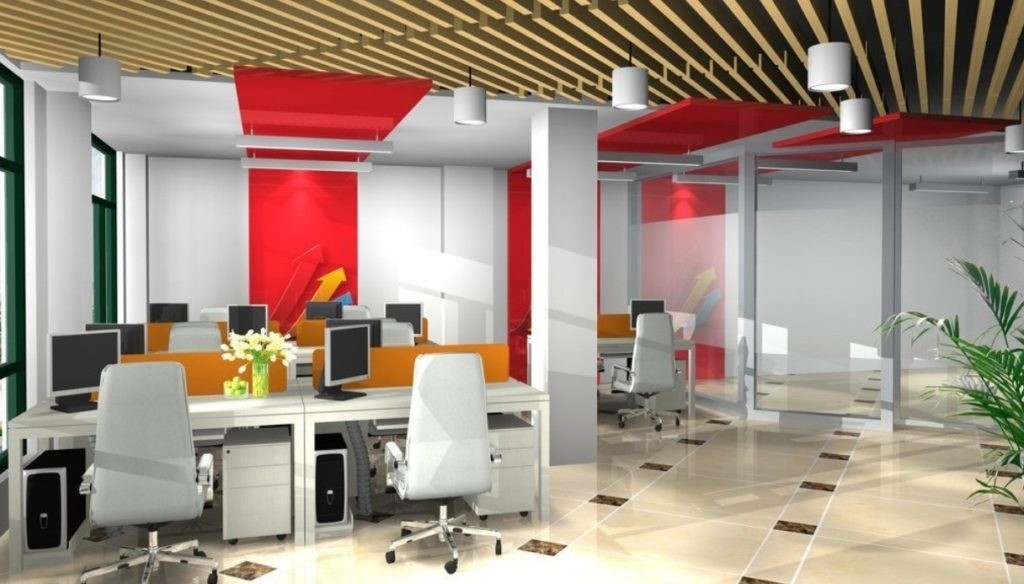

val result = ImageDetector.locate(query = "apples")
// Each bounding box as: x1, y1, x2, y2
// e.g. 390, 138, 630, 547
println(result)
224, 376, 248, 396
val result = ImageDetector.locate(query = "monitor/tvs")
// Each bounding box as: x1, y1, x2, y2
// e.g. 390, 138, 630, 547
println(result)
228, 304, 268, 338
306, 301, 423, 401
47, 303, 188, 413
630, 299, 665, 330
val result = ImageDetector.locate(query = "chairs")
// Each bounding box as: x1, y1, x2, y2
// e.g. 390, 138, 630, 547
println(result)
199, 308, 228, 322
78, 362, 220, 584
381, 321, 414, 347
609, 313, 680, 432
384, 351, 503, 572
169, 327, 223, 352
342, 305, 370, 319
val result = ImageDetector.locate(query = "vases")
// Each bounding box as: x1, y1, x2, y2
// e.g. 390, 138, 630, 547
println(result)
250, 360, 271, 396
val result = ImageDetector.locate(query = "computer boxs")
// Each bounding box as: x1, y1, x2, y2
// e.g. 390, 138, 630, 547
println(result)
22, 449, 87, 550
315, 432, 347, 526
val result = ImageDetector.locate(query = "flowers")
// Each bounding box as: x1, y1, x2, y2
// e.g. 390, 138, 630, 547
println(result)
219, 326, 300, 374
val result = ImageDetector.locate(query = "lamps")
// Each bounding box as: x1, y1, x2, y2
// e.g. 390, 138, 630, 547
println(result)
453, 67, 486, 125
841, 58, 872, 133
1005, 102, 1024, 152
611, 43, 649, 109
78, 34, 119, 102
807, 1, 851, 93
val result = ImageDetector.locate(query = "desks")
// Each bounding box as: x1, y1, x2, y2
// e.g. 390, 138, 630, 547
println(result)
597, 313, 697, 435
8, 320, 550, 571
